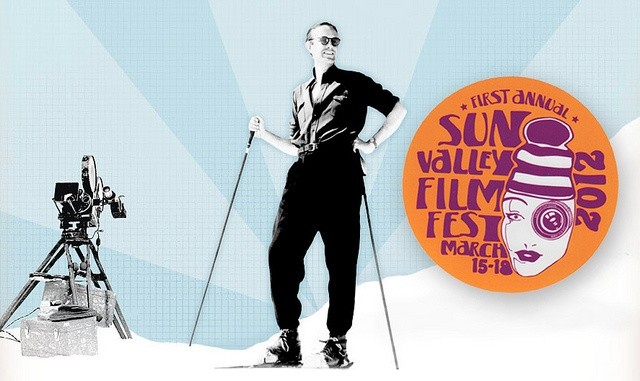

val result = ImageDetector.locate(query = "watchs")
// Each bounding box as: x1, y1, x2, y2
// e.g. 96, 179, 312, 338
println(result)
370, 137, 379, 151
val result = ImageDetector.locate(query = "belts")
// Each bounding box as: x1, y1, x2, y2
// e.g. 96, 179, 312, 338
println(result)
298, 143, 348, 153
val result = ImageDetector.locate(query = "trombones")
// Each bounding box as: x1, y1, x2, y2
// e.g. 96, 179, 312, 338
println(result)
215, 362, 355, 368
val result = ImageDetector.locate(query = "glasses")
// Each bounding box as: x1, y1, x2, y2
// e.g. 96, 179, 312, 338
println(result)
307, 37, 341, 47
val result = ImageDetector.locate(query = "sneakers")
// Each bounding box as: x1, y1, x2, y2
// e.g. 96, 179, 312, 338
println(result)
266, 331, 301, 362
314, 337, 347, 365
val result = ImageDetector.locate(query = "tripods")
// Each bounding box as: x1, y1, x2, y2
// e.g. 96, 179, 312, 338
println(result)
0, 229, 133, 339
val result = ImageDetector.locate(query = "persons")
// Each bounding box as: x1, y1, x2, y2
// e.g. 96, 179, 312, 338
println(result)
248, 20, 408, 368
